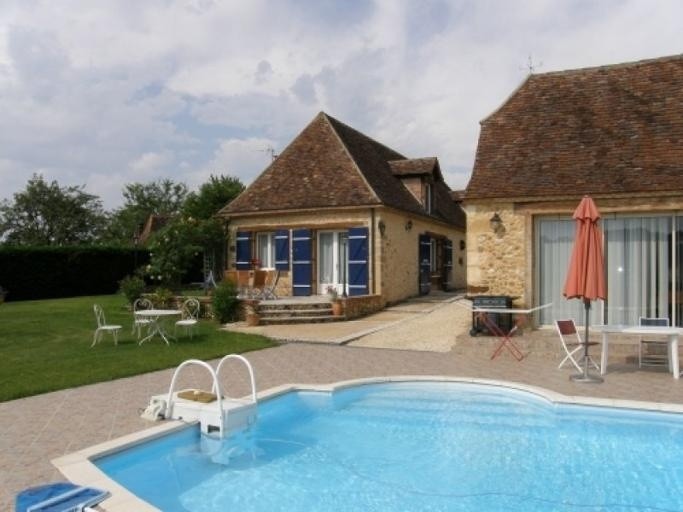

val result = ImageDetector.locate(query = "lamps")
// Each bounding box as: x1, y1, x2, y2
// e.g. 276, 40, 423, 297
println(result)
489, 212, 503, 234
405, 220, 415, 231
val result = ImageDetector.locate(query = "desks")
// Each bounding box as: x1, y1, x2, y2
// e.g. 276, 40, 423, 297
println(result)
132, 308, 182, 348
472, 304, 554, 362
589, 325, 683, 379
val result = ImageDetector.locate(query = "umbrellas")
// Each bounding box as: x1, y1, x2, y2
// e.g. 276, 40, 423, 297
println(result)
560, 190, 607, 335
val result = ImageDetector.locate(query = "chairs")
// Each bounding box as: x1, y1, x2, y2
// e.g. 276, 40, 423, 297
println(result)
638, 316, 672, 374
129, 298, 153, 340
174, 297, 201, 341
89, 303, 123, 347
205, 268, 282, 301
554, 320, 600, 374
